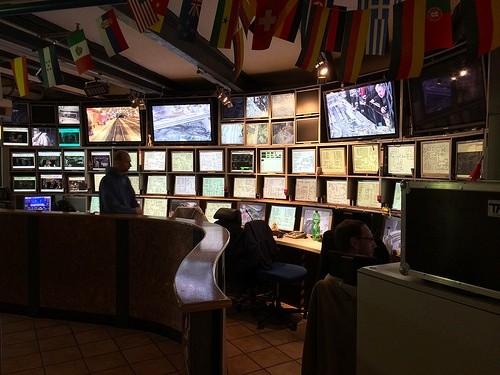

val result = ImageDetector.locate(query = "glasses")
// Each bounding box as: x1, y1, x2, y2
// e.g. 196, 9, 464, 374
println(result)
353, 236, 374, 242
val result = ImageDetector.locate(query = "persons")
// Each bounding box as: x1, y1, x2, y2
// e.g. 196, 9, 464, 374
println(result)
324, 220, 377, 298
99, 150, 143, 216
359, 84, 393, 126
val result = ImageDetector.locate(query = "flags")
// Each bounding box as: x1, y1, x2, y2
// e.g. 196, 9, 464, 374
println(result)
209, 0, 500, 83
67, 29, 94, 75
175, 0, 202, 42
97, 8, 129, 57
10, 57, 29, 97
38, 45, 65, 89
128, 0, 159, 34
146, 0, 169, 33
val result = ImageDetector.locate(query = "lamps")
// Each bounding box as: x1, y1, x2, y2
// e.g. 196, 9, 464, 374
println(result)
83, 76, 110, 97
315, 50, 331, 78
196, 68, 231, 92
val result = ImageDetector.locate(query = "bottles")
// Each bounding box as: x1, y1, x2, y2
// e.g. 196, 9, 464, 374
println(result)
384, 227, 393, 256
312, 210, 320, 238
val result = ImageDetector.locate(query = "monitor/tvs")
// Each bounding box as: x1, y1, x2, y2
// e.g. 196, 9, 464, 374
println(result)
4, 78, 486, 261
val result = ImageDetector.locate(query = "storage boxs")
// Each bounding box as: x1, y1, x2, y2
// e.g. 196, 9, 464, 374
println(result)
398, 176, 500, 299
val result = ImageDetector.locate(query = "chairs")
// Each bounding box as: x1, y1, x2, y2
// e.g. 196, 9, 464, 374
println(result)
241, 219, 308, 332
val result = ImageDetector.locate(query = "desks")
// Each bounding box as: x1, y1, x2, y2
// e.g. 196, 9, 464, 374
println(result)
354, 263, 500, 375
274, 232, 323, 255
